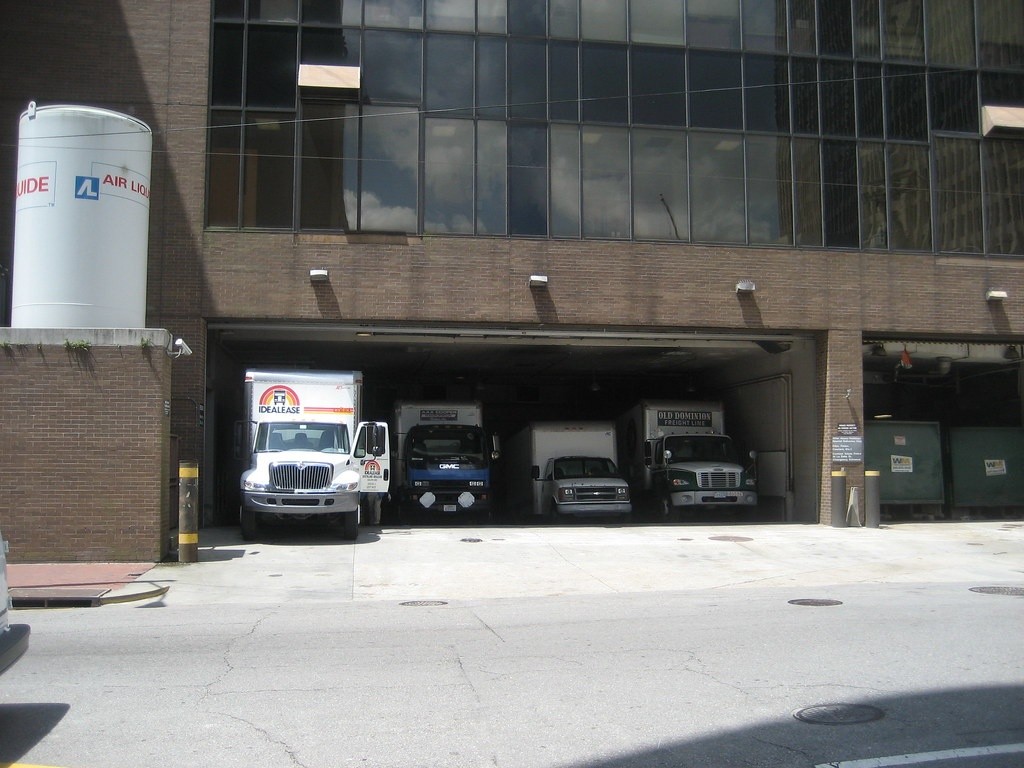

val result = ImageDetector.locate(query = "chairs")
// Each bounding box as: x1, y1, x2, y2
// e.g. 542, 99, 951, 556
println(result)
268, 432, 339, 451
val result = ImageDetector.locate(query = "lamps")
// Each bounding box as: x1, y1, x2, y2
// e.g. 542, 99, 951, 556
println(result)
310, 270, 330, 281
735, 282, 756, 294
1005, 347, 1021, 360
986, 290, 1008, 301
871, 343, 887, 357
530, 275, 548, 286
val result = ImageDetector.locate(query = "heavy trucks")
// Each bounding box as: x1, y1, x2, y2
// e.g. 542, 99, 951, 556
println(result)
505, 420, 633, 524
237, 367, 391, 542
390, 398, 501, 525
619, 396, 759, 522
